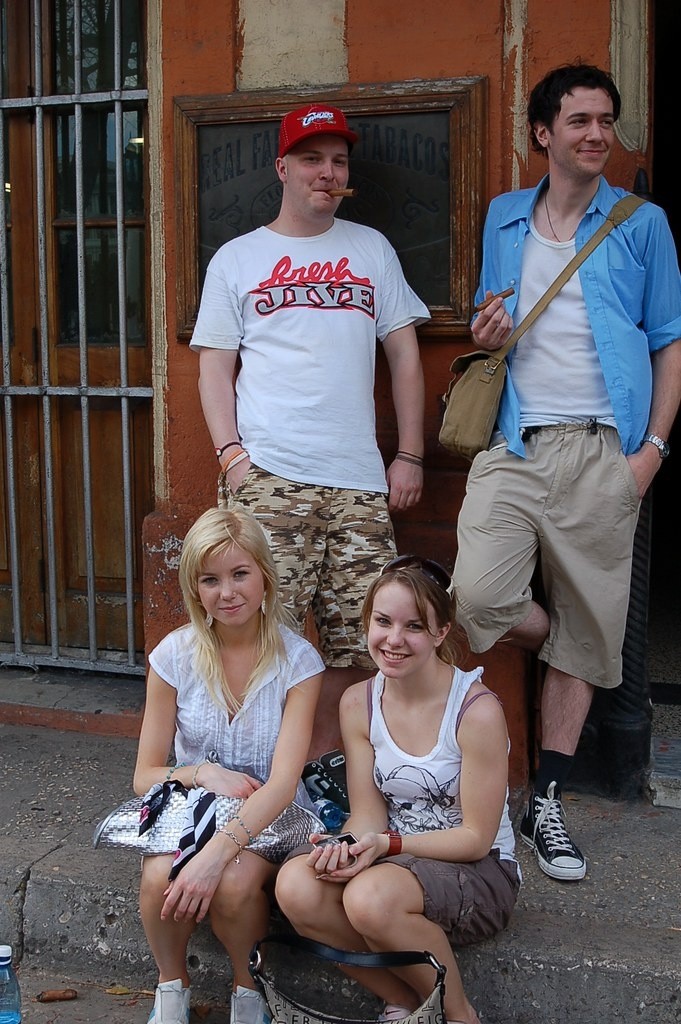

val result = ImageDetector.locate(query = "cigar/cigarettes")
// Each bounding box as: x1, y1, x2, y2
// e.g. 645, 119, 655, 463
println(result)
472, 287, 515, 316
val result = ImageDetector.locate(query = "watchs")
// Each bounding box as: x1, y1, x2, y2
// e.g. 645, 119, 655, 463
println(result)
644, 434, 671, 459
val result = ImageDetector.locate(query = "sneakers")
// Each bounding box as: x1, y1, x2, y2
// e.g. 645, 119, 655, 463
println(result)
229, 985, 273, 1024
146, 977, 192, 1024
300, 747, 352, 822
518, 786, 586, 880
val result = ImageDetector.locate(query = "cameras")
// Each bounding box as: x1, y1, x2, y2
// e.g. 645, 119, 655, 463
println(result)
313, 832, 359, 853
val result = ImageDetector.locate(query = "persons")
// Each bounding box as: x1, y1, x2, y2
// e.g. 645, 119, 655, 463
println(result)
188, 105, 432, 819
133, 506, 325, 1024
454, 62, 680, 880
275, 556, 522, 1024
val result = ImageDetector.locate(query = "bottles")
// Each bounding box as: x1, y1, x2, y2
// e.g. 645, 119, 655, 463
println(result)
308, 791, 345, 831
0, 945, 22, 1024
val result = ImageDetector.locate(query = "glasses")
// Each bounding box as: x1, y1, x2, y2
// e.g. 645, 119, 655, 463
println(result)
381, 554, 455, 602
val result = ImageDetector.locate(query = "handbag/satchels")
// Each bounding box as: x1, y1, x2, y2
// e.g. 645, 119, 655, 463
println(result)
438, 349, 507, 463
92, 749, 329, 865
247, 931, 447, 1024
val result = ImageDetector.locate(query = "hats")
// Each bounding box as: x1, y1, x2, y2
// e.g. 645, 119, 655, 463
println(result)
277, 106, 359, 159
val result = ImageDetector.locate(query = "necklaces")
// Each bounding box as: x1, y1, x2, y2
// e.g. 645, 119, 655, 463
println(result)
543, 194, 577, 243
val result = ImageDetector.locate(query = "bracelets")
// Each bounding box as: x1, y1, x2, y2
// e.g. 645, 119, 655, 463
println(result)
166, 761, 189, 781
380, 828, 402, 857
215, 442, 249, 474
213, 828, 243, 864
395, 449, 424, 468
191, 762, 207, 789
230, 815, 255, 845
